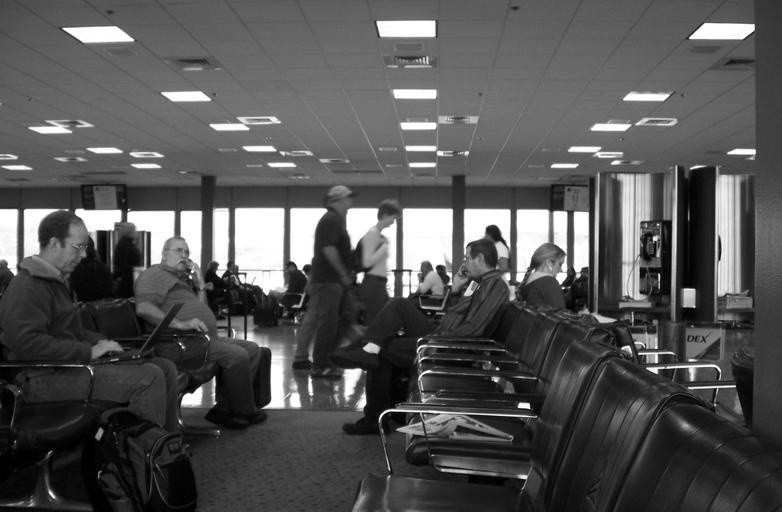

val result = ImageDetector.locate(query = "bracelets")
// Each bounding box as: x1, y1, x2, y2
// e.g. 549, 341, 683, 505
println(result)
337, 273, 349, 277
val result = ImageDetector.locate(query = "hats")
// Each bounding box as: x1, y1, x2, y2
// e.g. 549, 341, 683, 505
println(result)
327, 185, 360, 201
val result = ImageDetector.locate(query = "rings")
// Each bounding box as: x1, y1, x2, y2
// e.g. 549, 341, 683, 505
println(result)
109, 340, 114, 343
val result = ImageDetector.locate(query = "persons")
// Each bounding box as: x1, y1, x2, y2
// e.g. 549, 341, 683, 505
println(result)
360, 198, 402, 320
292, 284, 319, 370
1, 258, 13, 295
267, 262, 310, 320
515, 243, 566, 315
114, 221, 141, 298
138, 236, 266, 429
204, 260, 266, 321
329, 239, 510, 435
407, 261, 450, 308
560, 266, 590, 312
2, 210, 179, 433
307, 185, 356, 378
68, 236, 117, 303
485, 225, 511, 284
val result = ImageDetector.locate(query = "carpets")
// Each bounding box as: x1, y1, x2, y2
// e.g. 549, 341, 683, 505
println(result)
180, 405, 427, 512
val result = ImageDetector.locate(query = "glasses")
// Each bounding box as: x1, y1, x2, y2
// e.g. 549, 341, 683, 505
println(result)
72, 245, 89, 251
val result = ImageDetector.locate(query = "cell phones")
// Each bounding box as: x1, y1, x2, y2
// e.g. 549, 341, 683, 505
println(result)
185, 259, 192, 273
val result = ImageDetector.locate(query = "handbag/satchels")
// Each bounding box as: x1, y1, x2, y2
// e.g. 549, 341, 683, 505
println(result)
94, 407, 197, 512
353, 237, 383, 271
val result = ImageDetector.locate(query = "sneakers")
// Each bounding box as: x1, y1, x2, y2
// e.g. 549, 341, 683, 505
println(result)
204, 405, 268, 429
343, 418, 391, 435
292, 342, 380, 377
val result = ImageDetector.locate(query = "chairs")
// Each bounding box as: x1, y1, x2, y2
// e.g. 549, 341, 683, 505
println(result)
418, 286, 454, 313
0, 287, 242, 511
279, 284, 309, 310
350, 339, 782, 511
403, 301, 739, 482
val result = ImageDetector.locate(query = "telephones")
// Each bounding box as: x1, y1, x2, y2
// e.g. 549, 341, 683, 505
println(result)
641, 233, 652, 261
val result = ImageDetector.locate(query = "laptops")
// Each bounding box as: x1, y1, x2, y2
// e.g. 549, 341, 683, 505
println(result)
90, 302, 184, 366
